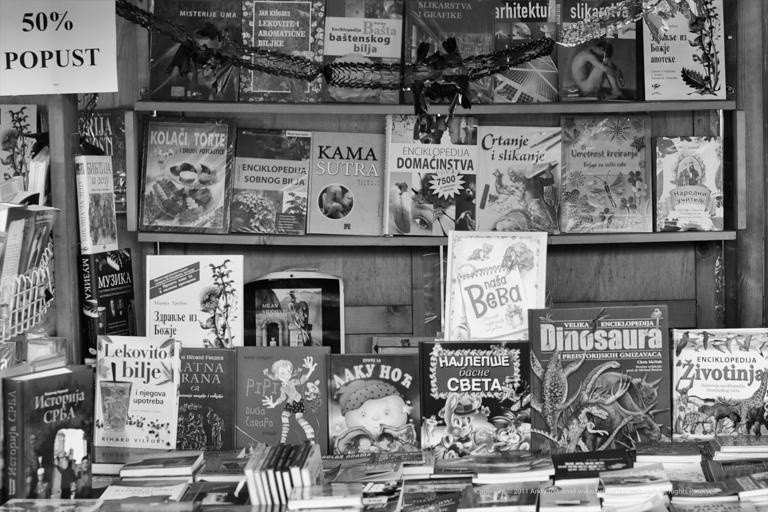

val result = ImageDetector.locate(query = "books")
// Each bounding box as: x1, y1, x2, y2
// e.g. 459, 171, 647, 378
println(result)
141, 1, 732, 103
2, 103, 768, 512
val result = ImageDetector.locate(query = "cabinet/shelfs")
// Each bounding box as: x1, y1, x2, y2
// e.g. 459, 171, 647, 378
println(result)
121, 95, 747, 246
